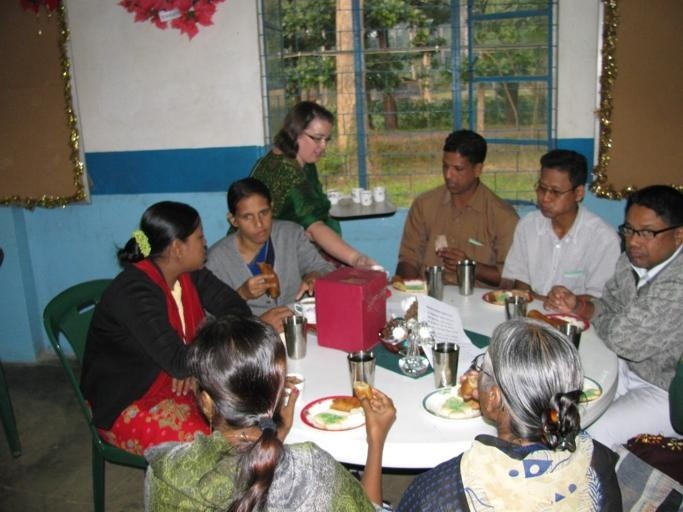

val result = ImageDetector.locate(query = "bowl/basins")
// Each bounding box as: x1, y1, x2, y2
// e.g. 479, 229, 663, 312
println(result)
283, 373, 305, 393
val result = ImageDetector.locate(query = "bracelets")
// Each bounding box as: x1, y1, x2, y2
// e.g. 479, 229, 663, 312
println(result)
347, 252, 368, 266
572, 297, 586, 317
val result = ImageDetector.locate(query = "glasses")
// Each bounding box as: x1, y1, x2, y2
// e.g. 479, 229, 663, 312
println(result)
472, 353, 491, 377
305, 133, 331, 143
538, 184, 579, 196
619, 224, 680, 239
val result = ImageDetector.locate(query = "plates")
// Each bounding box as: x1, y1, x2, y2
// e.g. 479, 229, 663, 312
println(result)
422, 387, 481, 419
387, 279, 426, 295
578, 376, 603, 404
545, 314, 590, 332
300, 395, 365, 431
482, 290, 534, 307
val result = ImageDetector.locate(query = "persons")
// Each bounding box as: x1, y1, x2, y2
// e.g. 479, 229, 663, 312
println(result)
500, 148, 622, 306
79, 199, 295, 457
140, 311, 397, 512
541, 183, 683, 389
394, 126, 522, 289
225, 100, 385, 276
395, 312, 623, 511
205, 177, 337, 321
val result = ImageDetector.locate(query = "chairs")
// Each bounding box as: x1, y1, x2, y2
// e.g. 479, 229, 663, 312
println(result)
0, 246, 21, 459
43, 279, 151, 511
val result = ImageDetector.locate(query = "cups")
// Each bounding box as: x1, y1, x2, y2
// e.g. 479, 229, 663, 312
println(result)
456, 259, 476, 295
294, 297, 317, 325
504, 295, 528, 319
328, 191, 342, 205
281, 316, 307, 360
351, 187, 386, 206
431, 342, 461, 389
347, 350, 377, 398
559, 325, 582, 352
425, 265, 446, 300
